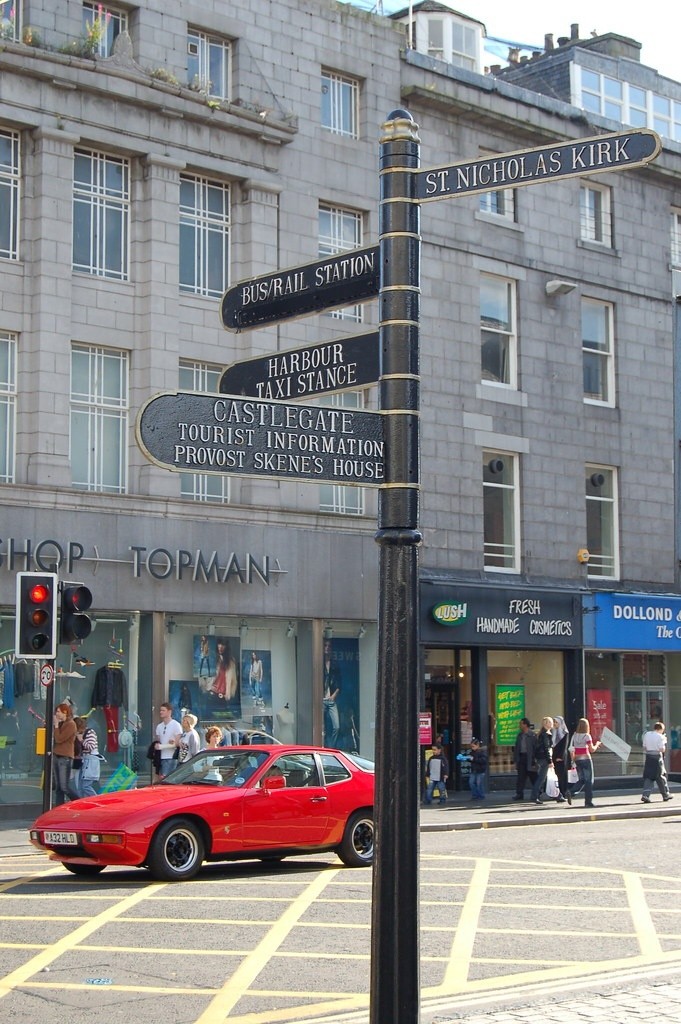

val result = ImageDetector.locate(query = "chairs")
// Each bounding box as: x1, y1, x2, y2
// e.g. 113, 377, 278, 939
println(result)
286, 770, 308, 787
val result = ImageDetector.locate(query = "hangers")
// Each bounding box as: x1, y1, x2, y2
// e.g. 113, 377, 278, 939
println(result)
0, 650, 28, 665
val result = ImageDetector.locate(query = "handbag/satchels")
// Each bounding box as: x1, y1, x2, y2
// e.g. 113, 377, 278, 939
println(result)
81, 755, 101, 781
546, 764, 559, 797
567, 763, 579, 783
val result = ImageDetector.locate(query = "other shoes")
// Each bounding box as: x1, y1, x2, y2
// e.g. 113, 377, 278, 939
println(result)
421, 801, 431, 806
586, 802, 599, 808
513, 792, 524, 801
641, 796, 651, 803
536, 799, 544, 804
437, 801, 446, 805
567, 790, 572, 805
557, 796, 566, 802
471, 798, 480, 801
663, 794, 673, 800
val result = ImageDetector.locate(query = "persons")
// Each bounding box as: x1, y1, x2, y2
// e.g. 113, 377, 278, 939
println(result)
530, 717, 566, 804
179, 713, 200, 766
197, 636, 237, 719
249, 652, 265, 707
566, 718, 601, 807
53, 703, 80, 806
200, 636, 212, 677
551, 716, 570, 797
422, 743, 449, 804
466, 739, 487, 800
512, 718, 537, 800
323, 638, 340, 748
177, 684, 192, 710
193, 726, 224, 783
641, 722, 674, 803
155, 704, 183, 776
73, 717, 99, 798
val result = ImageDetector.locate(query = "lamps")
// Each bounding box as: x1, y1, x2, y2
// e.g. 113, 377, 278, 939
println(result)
206, 618, 216, 636
239, 619, 248, 636
358, 623, 368, 639
166, 616, 178, 634
324, 622, 334, 639
545, 280, 578, 296
285, 621, 296, 638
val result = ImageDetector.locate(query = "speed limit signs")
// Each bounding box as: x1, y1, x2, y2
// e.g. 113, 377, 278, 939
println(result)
40, 664, 54, 686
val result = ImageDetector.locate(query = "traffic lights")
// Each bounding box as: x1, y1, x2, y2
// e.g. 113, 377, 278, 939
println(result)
15, 571, 57, 659
60, 580, 92, 645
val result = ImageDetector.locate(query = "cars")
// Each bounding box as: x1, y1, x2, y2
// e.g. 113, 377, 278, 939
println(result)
28, 744, 375, 882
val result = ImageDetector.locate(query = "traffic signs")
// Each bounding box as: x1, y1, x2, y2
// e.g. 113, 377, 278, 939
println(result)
219, 243, 381, 335
217, 331, 383, 403
413, 127, 665, 206
134, 389, 384, 489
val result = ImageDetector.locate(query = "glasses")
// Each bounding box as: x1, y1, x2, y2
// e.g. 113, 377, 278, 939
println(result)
163, 726, 166, 735
